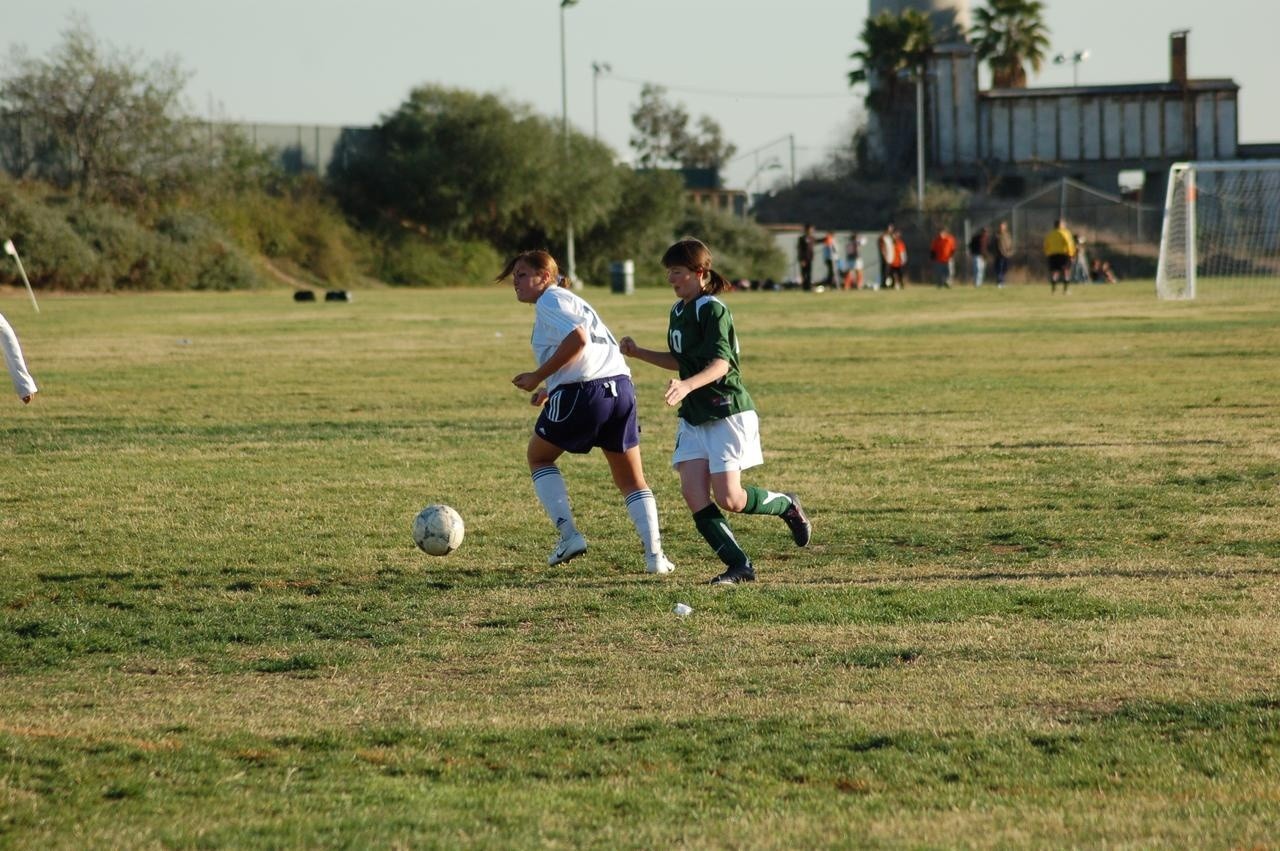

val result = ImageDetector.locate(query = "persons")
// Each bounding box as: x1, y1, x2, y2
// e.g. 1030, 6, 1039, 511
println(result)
619, 238, 812, 584
0, 313, 38, 404
796, 218, 1117, 297
493, 250, 675, 575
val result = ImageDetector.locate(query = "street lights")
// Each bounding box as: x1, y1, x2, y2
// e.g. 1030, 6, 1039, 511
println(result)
558, 0, 580, 286
1052, 52, 1091, 83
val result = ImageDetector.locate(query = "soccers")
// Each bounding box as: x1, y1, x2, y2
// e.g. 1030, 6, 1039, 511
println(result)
412, 504, 465, 556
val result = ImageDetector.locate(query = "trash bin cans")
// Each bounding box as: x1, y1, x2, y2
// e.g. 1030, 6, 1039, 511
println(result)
611, 260, 633, 293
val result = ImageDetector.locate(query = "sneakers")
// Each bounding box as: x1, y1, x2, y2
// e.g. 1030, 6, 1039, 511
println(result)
779, 493, 812, 547
647, 551, 676, 574
550, 532, 588, 566
711, 565, 760, 590
